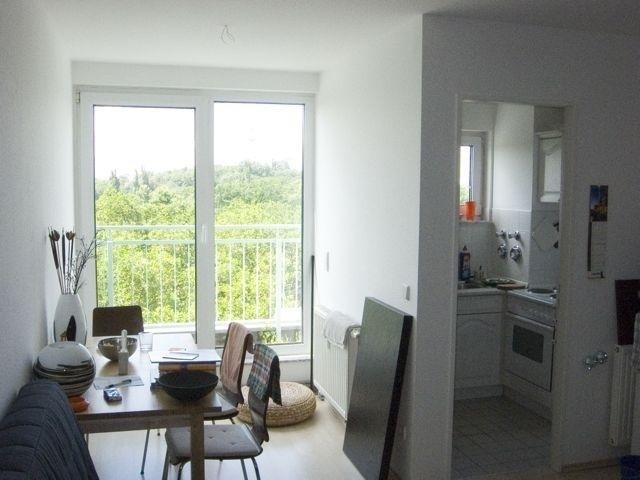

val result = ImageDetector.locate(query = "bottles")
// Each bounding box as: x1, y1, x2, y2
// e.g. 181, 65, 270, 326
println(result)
117, 329, 130, 376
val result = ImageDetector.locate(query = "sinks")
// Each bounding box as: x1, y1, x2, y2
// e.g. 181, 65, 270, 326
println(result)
457, 279, 488, 290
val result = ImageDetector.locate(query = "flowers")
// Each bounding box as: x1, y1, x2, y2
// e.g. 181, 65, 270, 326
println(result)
46, 225, 107, 294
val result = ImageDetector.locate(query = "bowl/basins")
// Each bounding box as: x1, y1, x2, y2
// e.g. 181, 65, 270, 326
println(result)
97, 336, 138, 362
157, 368, 220, 399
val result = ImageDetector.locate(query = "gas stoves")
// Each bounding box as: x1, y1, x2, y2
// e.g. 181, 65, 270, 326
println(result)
506, 287, 557, 326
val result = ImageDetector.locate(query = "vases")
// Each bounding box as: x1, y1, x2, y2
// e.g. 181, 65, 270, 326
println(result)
54, 292, 89, 345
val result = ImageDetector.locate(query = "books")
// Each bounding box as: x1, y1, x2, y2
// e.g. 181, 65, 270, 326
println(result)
147, 348, 223, 377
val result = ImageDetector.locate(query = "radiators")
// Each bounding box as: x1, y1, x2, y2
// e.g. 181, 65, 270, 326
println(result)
311, 305, 361, 426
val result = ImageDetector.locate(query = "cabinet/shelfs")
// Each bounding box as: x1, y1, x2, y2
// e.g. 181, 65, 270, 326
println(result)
454, 295, 504, 389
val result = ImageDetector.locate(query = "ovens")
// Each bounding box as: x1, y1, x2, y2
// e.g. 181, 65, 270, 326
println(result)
497, 311, 556, 393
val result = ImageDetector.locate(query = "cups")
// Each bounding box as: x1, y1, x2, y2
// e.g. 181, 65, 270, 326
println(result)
138, 331, 153, 351
465, 202, 476, 221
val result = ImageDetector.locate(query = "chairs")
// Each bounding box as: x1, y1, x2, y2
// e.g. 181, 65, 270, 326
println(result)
140, 323, 278, 480
91, 304, 145, 337
1, 378, 100, 478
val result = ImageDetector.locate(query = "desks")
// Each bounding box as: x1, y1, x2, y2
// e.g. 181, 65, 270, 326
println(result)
75, 333, 216, 479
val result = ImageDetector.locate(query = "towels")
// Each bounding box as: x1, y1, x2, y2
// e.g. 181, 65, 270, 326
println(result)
319, 309, 359, 350
